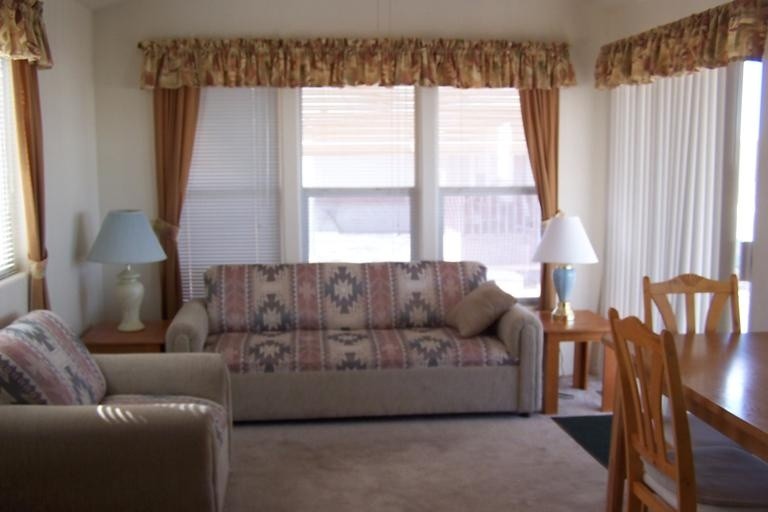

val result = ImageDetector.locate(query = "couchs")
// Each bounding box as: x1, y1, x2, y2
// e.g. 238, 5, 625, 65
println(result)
164, 260, 545, 423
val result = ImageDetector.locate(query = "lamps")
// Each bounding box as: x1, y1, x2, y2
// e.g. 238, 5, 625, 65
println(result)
533, 215, 600, 321
84, 208, 169, 332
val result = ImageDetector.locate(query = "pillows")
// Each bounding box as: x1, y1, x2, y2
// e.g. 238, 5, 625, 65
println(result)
0, 309, 108, 405
448, 280, 517, 338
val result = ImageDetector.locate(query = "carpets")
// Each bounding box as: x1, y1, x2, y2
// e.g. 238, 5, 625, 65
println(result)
550, 416, 611, 469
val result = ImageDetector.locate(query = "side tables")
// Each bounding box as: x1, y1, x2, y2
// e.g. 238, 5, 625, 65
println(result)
80, 320, 170, 354
529, 310, 616, 415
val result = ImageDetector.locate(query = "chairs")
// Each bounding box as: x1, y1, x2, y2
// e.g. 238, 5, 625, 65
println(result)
642, 273, 742, 449
0, 352, 235, 512
608, 307, 768, 512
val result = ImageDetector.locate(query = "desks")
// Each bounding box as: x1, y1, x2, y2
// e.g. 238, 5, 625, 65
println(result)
600, 331, 768, 512
